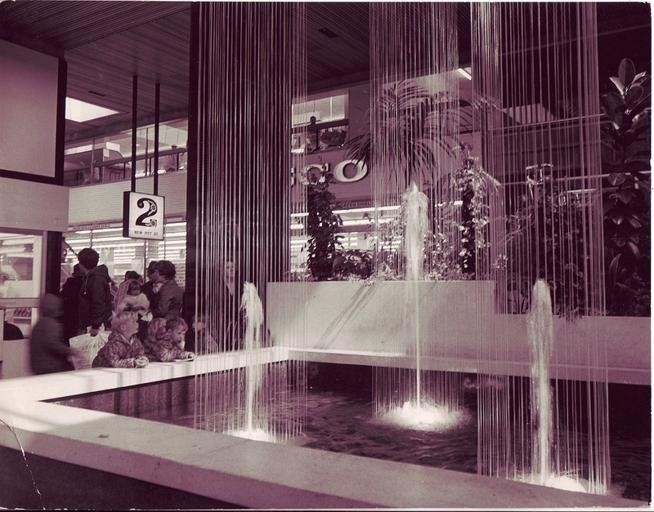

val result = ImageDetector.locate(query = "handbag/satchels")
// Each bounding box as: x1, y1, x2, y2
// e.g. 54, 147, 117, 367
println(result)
68, 331, 112, 369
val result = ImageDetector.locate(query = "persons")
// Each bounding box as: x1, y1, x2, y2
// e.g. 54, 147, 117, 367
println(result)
208, 257, 247, 351
166, 145, 181, 172
303, 116, 322, 153
27, 247, 195, 376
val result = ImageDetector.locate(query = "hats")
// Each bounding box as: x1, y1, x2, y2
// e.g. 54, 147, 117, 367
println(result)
144, 261, 159, 270
